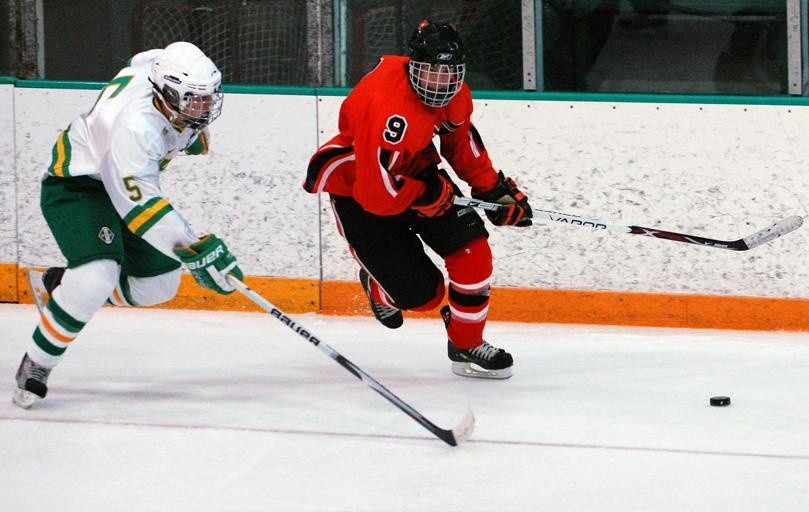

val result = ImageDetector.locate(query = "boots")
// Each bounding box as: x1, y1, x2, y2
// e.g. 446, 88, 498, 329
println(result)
15, 352, 52, 399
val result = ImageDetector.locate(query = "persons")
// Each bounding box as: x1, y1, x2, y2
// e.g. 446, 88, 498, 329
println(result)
14, 39, 245, 400
303, 17, 534, 369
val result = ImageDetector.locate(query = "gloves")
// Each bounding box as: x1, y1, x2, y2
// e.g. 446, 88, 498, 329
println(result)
409, 171, 455, 220
173, 233, 243, 294
471, 169, 533, 227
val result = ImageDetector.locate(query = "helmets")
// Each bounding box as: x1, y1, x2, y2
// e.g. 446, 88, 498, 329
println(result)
148, 41, 222, 117
409, 22, 467, 108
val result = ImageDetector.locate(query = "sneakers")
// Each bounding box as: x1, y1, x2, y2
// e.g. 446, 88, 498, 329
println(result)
359, 267, 403, 329
448, 337, 513, 370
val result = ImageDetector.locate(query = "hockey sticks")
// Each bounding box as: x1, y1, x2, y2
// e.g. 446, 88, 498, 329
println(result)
225, 275, 476, 447
454, 197, 803, 253
